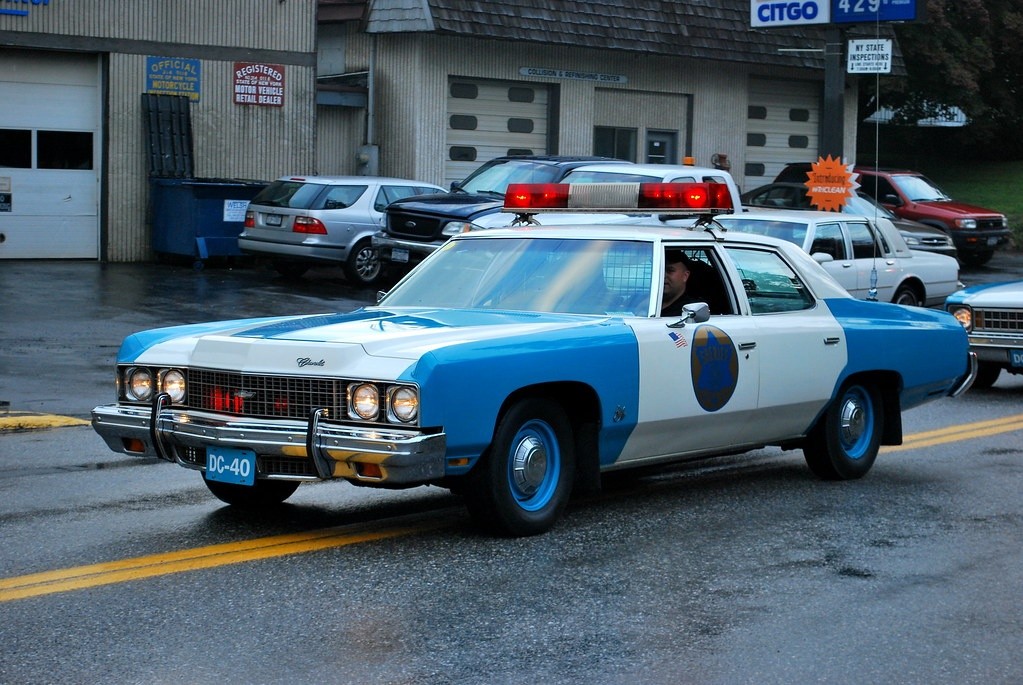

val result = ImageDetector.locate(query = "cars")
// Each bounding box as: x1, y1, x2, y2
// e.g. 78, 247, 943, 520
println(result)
739, 178, 958, 259
702, 208, 965, 310
237, 175, 450, 291
90, 181, 979, 540
945, 279, 1023, 390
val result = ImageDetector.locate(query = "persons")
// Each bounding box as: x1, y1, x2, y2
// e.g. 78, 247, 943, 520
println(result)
623, 250, 710, 316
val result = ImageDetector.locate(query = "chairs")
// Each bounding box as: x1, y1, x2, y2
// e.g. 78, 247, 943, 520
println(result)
519, 245, 618, 312
625, 256, 733, 317
767, 224, 793, 242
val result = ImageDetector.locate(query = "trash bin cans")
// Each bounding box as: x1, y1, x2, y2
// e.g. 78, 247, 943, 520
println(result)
141, 92, 273, 271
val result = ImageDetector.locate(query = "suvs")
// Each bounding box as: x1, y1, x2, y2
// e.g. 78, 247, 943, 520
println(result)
768, 161, 1012, 266
370, 155, 638, 277
468, 162, 742, 230
